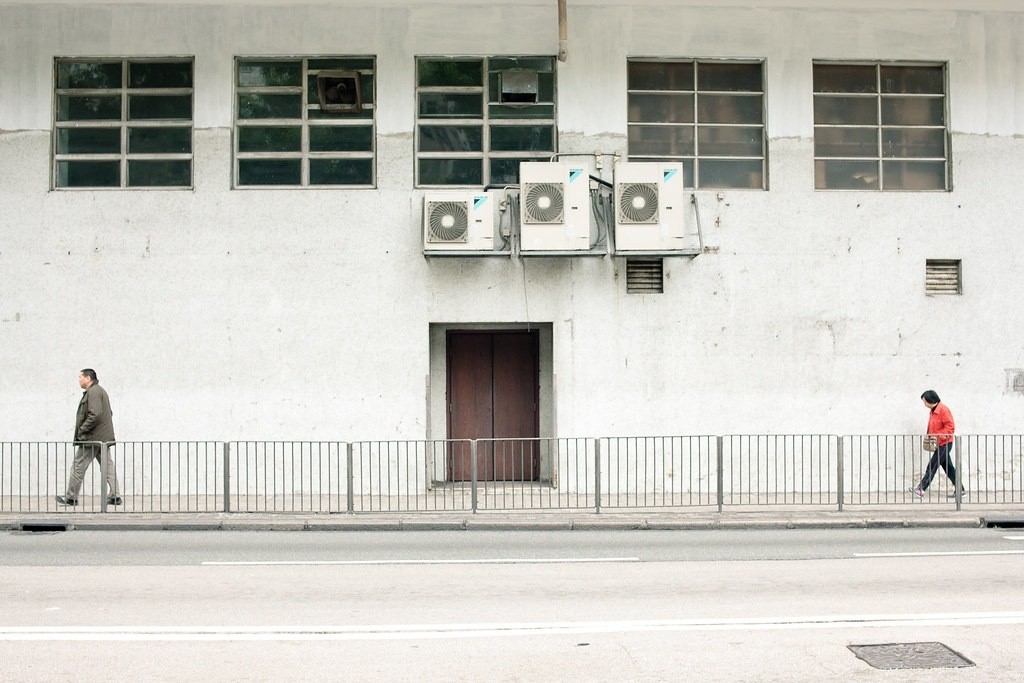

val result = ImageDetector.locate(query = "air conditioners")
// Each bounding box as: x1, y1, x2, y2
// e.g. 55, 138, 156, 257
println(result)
423, 194, 493, 251
520, 161, 590, 251
612, 160, 683, 250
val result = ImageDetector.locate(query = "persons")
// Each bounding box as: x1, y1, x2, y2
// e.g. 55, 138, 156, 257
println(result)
909, 390, 965, 498
56, 369, 123, 505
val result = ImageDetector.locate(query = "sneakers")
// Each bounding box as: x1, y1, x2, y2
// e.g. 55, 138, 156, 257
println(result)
948, 489, 966, 498
908, 486, 925, 498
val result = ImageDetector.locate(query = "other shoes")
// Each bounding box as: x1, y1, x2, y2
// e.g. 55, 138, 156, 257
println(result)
55, 495, 77, 505
106, 497, 122, 505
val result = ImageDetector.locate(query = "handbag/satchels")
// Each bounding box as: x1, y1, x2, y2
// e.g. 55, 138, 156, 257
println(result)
923, 435, 938, 452
